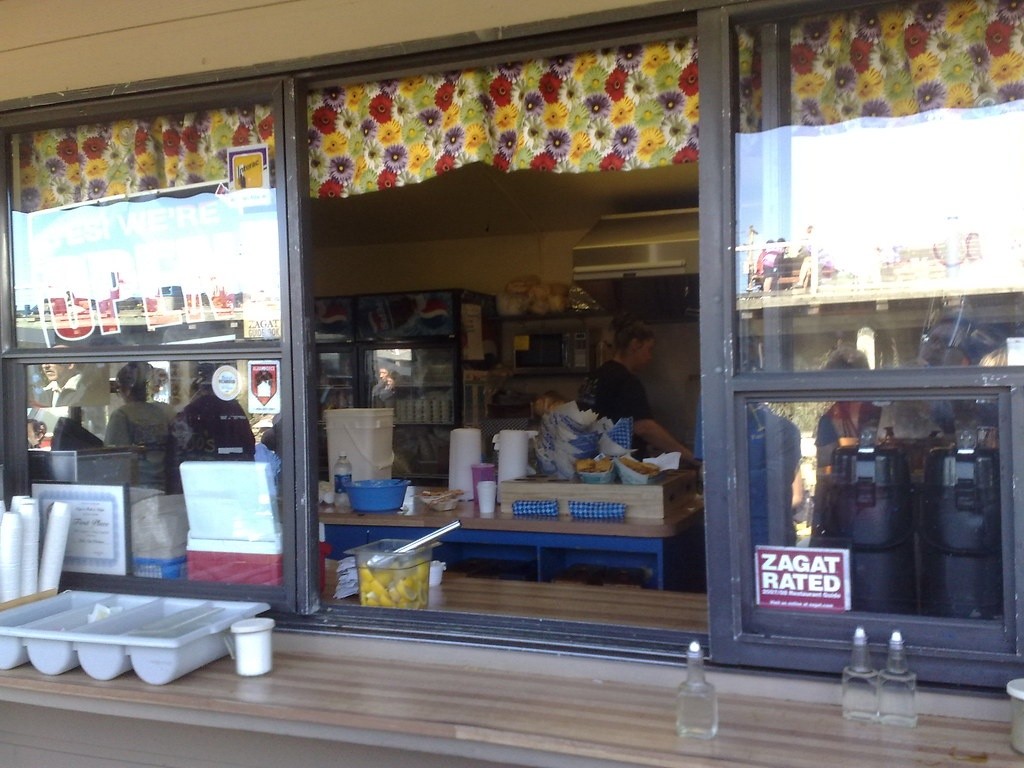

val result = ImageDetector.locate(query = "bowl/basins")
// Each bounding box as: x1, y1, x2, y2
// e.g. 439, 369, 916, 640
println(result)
343, 480, 411, 514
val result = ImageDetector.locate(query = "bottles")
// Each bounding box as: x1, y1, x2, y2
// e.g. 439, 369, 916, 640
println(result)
333, 451, 353, 507
841, 627, 879, 723
879, 630, 918, 729
675, 641, 719, 740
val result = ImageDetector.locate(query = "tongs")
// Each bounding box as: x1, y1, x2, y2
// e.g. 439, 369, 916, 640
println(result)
367, 520, 462, 569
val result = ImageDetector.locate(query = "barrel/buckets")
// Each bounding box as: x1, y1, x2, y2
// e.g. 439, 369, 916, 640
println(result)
324, 408, 395, 483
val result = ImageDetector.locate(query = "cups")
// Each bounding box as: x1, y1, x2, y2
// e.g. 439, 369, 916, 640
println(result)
476, 481, 497, 513
470, 462, 494, 512
230, 618, 277, 677
343, 537, 442, 609
1007, 678, 1024, 755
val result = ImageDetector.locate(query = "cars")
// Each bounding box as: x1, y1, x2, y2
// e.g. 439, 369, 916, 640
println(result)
30, 298, 50, 315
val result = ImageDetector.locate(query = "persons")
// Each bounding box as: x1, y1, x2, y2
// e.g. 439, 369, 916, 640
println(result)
579, 323, 696, 465
371, 364, 411, 409
24, 364, 281, 494
753, 349, 881, 551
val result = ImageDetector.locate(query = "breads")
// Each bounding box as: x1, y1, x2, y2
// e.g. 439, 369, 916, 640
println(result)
505, 280, 567, 313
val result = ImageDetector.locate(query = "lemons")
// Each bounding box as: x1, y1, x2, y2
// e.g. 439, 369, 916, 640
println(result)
358, 559, 430, 612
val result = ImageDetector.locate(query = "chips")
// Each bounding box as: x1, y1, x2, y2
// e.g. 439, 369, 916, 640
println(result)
575, 456, 611, 473
